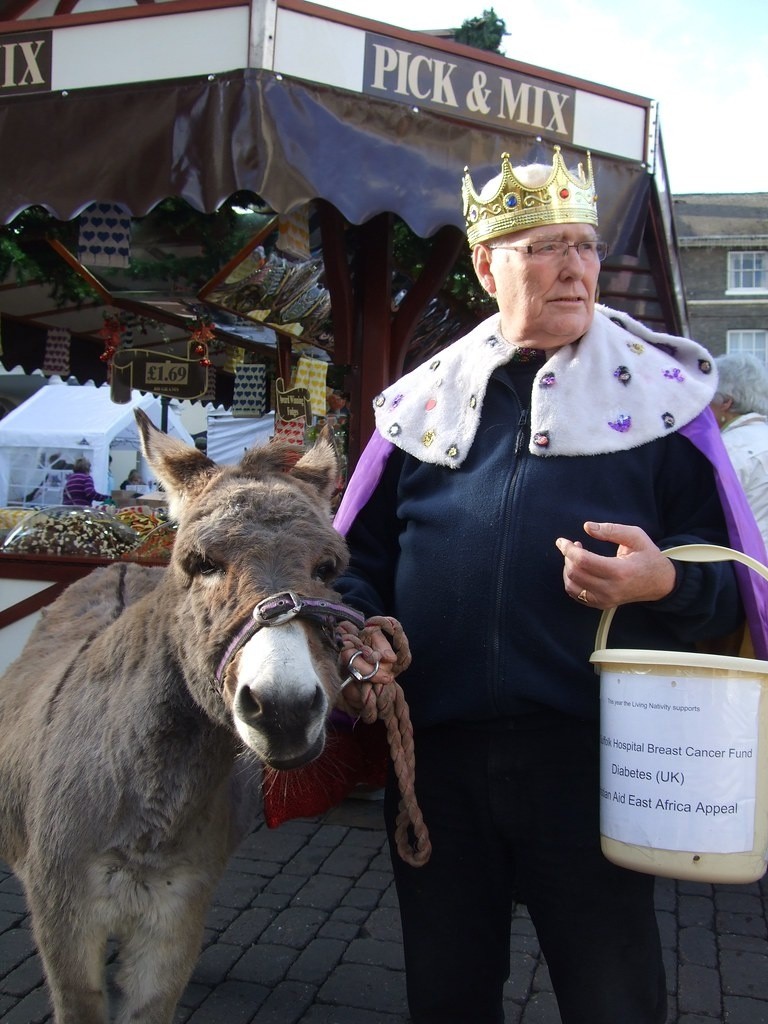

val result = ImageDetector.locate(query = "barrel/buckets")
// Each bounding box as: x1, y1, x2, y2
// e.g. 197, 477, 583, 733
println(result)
589, 544, 768, 884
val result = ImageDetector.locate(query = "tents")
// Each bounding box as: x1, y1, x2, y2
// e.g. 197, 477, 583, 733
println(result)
207, 406, 316, 474
0, 385, 196, 507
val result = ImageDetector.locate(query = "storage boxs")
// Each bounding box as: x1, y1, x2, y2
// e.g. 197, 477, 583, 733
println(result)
111, 485, 169, 508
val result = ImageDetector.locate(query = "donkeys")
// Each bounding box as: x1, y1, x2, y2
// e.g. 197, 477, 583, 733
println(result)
0, 409, 350, 1024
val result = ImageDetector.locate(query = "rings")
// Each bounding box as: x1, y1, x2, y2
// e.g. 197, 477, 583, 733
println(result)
577, 589, 588, 603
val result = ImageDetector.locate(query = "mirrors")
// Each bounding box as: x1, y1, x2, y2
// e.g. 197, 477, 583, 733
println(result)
44, 190, 478, 377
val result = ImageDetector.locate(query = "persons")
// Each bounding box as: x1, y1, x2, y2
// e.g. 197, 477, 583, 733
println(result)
62, 459, 111, 508
121, 469, 146, 490
48, 454, 74, 487
322, 143, 768, 1024
711, 352, 768, 557
109, 455, 115, 495
